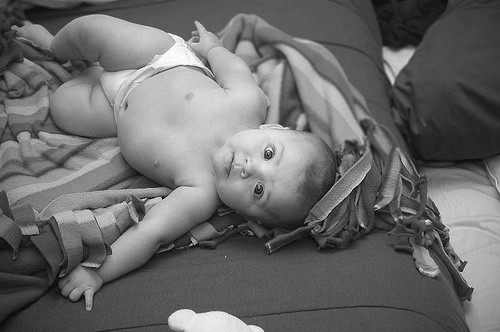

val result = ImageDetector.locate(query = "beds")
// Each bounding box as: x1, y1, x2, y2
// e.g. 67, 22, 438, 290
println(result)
1, 0, 470, 332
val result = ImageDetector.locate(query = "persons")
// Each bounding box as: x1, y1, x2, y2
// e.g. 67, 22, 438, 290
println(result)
9, 13, 336, 311
